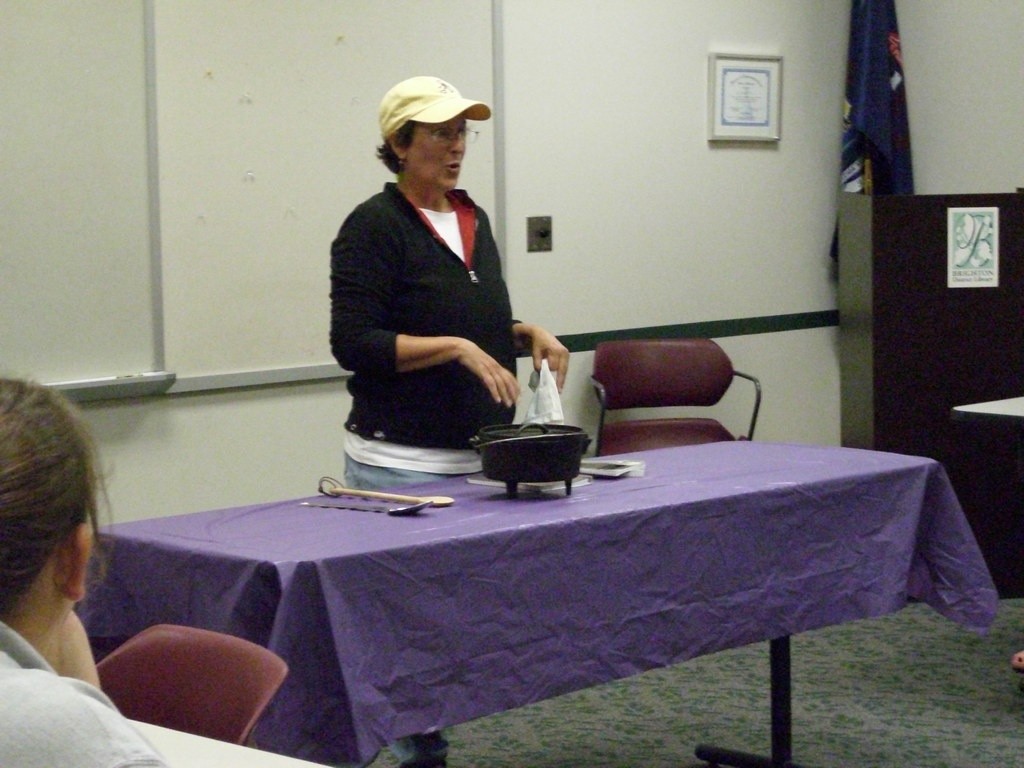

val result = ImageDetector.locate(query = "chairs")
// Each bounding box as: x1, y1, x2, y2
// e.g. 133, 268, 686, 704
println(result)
591, 339, 762, 460
96, 628, 288, 746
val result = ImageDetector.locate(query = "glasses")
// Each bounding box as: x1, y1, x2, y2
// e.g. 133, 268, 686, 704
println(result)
412, 127, 479, 147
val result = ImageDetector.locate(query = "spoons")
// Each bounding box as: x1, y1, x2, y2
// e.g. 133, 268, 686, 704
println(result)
298, 500, 433, 516
329, 488, 456, 507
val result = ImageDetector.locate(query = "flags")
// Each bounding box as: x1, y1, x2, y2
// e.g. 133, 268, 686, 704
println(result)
829, 0, 913, 264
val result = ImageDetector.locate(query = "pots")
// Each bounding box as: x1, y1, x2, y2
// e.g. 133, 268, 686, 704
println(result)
470, 423, 589, 499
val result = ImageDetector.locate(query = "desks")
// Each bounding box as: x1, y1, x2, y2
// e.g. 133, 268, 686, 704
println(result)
67, 441, 1001, 768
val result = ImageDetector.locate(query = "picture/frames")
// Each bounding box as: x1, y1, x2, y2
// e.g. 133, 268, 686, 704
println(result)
709, 52, 783, 142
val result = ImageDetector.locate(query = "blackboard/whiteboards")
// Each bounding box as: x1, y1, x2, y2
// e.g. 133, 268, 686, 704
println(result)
1, 0, 508, 404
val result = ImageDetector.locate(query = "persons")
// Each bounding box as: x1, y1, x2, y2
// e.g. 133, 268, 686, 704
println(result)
329, 75, 569, 767
0, 377, 160, 768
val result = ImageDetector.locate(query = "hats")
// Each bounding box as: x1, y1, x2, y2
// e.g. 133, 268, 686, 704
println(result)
378, 76, 491, 141
